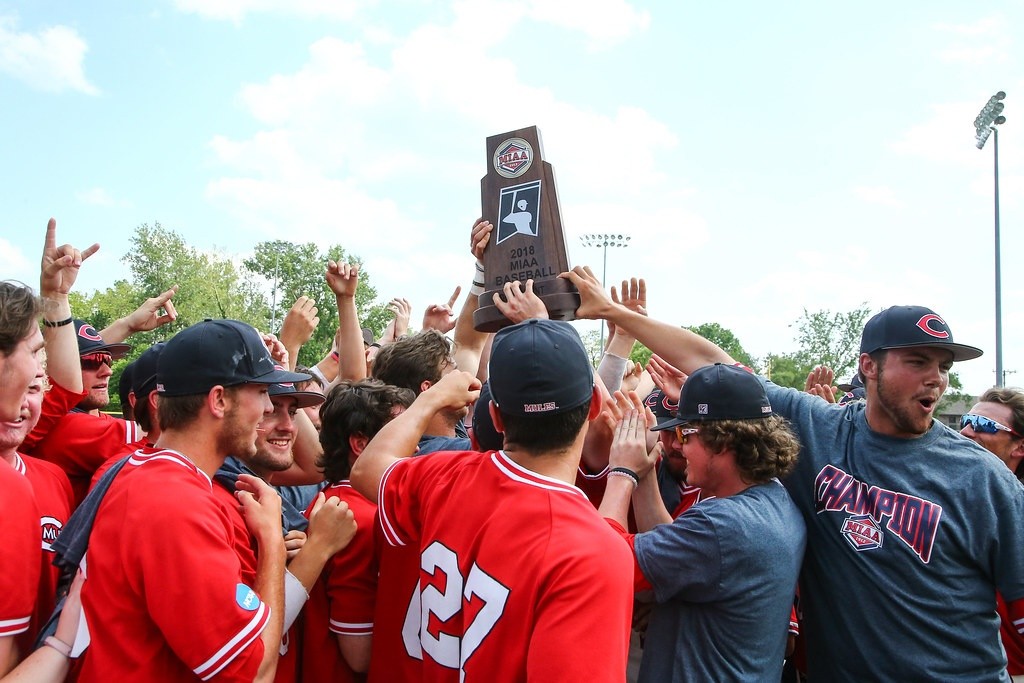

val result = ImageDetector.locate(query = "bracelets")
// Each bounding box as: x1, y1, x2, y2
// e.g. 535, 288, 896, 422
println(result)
43, 636, 73, 657
607, 466, 639, 490
43, 317, 73, 327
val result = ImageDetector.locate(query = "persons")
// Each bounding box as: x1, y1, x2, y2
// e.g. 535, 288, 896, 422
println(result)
597, 363, 807, 683
349, 318, 634, 683
558, 265, 1024, 683
0, 280, 86, 683
0, 347, 76, 658
77, 319, 312, 683
958, 386, 1024, 485
18, 217, 867, 683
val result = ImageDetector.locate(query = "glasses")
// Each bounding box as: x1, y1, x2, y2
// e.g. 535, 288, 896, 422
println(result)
80, 351, 113, 370
959, 413, 1022, 440
674, 427, 700, 445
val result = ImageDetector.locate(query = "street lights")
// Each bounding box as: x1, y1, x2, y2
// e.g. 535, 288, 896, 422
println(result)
974, 89, 1007, 387
579, 233, 631, 357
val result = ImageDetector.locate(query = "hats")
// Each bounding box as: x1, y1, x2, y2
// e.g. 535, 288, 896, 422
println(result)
472, 384, 504, 452
650, 362, 774, 431
155, 318, 314, 397
119, 339, 169, 403
641, 388, 680, 431
71, 320, 133, 356
267, 357, 327, 409
836, 372, 865, 405
489, 317, 593, 416
860, 305, 983, 362
